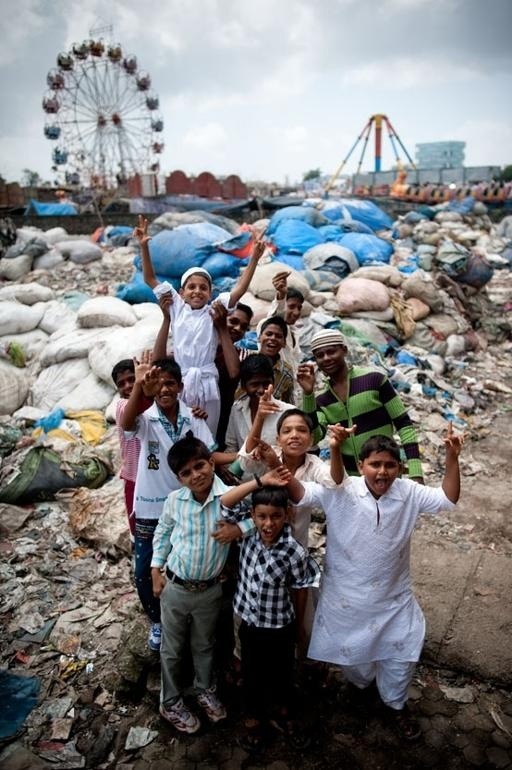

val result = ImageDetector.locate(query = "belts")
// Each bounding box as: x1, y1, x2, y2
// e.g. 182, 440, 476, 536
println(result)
165, 566, 224, 594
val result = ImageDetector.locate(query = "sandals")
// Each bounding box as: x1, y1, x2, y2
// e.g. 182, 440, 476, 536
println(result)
384, 703, 422, 740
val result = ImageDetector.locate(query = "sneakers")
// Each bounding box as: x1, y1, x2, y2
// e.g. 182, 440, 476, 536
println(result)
150, 622, 162, 652
159, 684, 228, 735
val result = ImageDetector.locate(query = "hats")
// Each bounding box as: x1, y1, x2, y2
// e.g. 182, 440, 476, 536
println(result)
309, 328, 345, 352
180, 267, 213, 288
